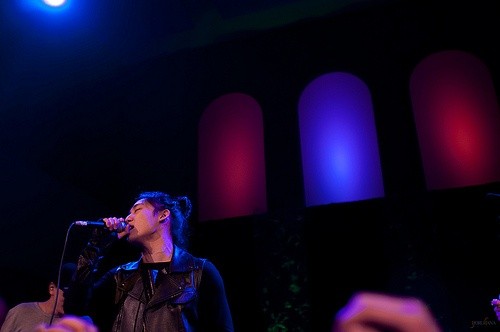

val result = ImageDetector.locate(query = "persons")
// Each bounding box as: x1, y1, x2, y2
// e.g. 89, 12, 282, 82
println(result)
0, 262, 78, 332
63, 192, 234, 332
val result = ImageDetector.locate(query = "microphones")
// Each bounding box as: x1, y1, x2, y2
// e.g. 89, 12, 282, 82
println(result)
76, 221, 126, 231
